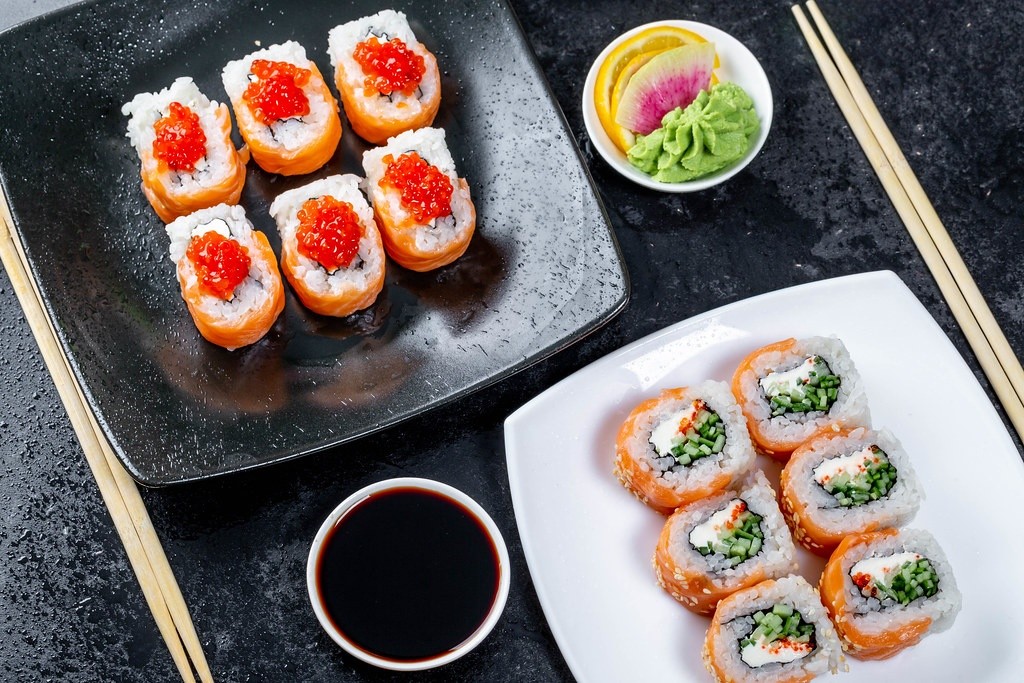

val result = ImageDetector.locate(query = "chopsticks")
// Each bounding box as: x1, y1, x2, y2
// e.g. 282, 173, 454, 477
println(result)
0, 194, 213, 683
791, 0, 1024, 448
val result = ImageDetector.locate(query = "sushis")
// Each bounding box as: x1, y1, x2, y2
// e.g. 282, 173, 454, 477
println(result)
119, 8, 475, 351
612, 335, 954, 682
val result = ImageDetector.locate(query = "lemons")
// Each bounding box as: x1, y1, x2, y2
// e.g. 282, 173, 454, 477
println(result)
593, 26, 718, 157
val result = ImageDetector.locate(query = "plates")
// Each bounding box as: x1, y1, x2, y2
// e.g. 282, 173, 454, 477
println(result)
580, 16, 777, 193
300, 474, 514, 671
502, 265, 1021, 682
1, 0, 630, 491
